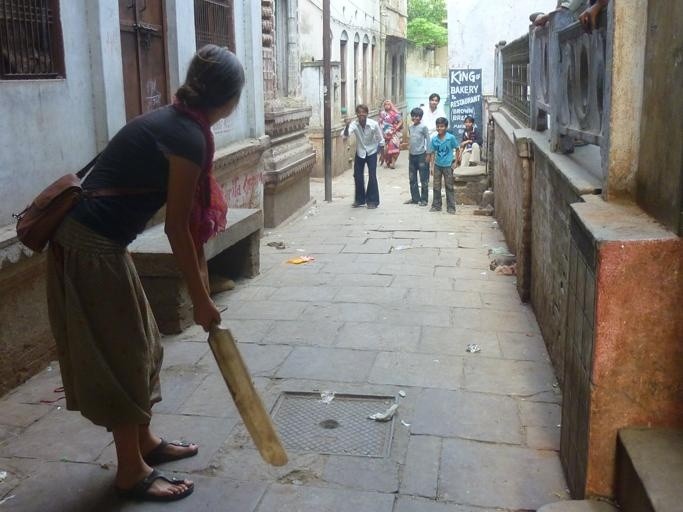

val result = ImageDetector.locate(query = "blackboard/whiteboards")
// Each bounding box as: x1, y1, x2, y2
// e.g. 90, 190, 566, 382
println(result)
449, 69, 483, 139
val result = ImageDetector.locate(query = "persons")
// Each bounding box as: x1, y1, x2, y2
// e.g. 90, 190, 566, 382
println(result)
458, 116, 483, 162
577, 1, 609, 35
534, 1, 588, 27
405, 107, 431, 205
45, 43, 246, 501
418, 94, 445, 186
340, 104, 384, 209
379, 101, 403, 169
429, 117, 460, 213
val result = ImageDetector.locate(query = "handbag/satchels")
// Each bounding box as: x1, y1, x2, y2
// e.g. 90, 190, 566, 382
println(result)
16, 172, 85, 253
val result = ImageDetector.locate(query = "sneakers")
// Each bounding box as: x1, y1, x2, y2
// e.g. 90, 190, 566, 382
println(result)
402, 197, 429, 206
351, 199, 379, 209
429, 205, 442, 212
447, 206, 456, 214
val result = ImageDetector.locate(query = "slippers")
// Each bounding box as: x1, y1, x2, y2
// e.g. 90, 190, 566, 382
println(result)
139, 435, 200, 466
114, 467, 196, 502
